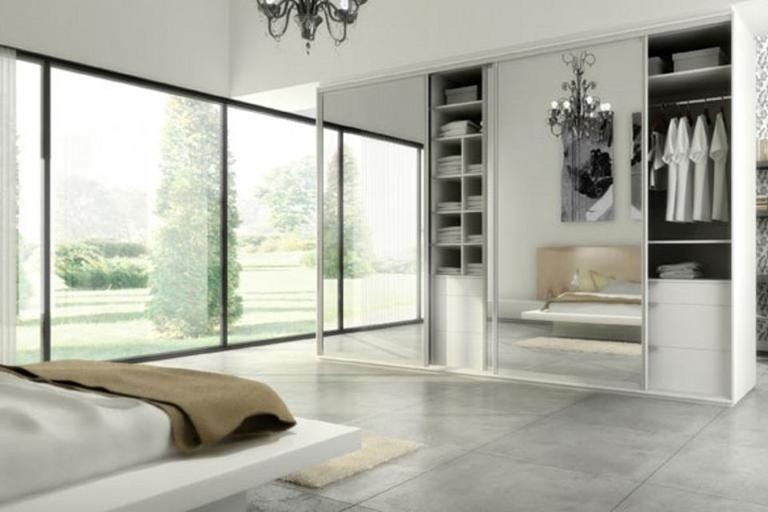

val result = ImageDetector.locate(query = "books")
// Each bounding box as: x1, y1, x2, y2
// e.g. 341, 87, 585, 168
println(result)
436, 120, 483, 275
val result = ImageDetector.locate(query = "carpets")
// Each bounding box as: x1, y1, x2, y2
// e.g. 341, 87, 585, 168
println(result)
272, 424, 424, 487
513, 335, 643, 357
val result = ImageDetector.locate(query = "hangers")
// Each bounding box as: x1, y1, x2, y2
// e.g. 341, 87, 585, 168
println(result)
648, 95, 729, 141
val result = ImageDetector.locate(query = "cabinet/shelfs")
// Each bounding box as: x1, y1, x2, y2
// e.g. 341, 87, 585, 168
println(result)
312, 5, 758, 408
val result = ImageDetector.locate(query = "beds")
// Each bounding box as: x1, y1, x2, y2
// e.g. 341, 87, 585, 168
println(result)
1, 348, 364, 512
520, 238, 644, 342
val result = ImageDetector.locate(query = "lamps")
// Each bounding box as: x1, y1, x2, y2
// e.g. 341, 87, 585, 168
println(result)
255, 2, 365, 53
543, 51, 616, 141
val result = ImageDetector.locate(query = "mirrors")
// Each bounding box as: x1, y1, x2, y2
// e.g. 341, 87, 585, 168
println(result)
481, 33, 646, 393
314, 70, 429, 376
